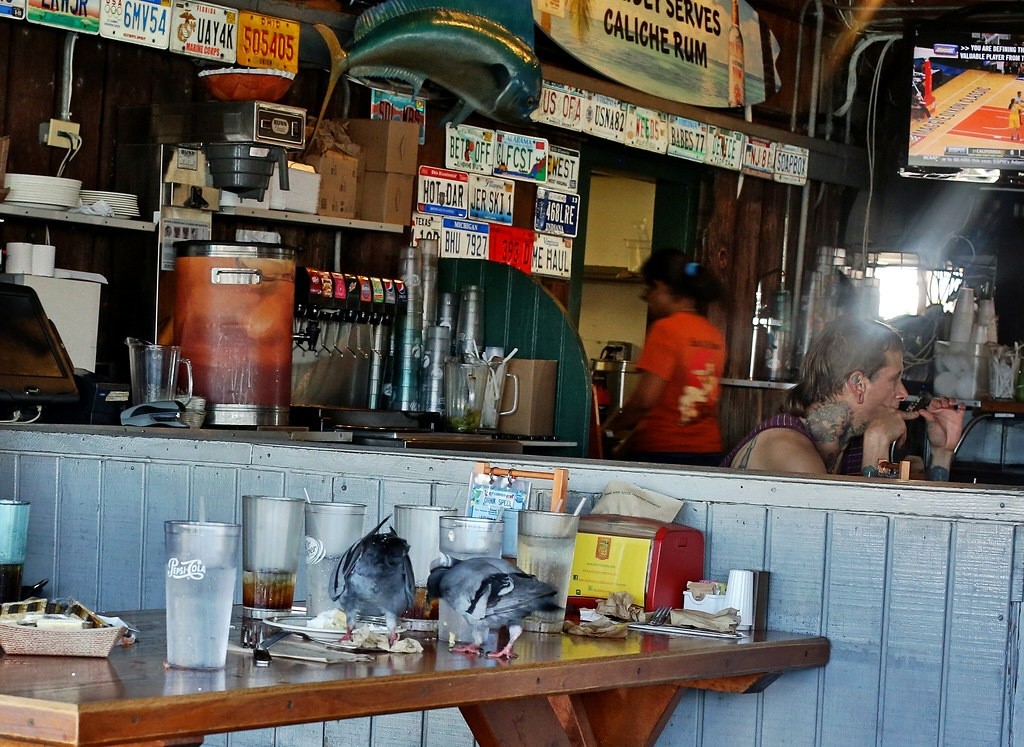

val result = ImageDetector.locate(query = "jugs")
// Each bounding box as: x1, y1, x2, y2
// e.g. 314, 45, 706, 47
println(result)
126, 336, 193, 406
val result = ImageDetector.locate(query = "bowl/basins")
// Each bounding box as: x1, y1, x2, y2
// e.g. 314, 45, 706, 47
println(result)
200, 73, 294, 102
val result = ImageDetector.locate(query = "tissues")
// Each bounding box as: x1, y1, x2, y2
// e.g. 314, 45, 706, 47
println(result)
563, 481, 708, 616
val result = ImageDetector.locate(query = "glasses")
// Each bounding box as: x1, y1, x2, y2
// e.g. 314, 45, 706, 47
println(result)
640, 285, 655, 302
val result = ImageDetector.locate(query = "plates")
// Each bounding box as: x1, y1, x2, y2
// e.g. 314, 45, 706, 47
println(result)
4, 172, 140, 221
264, 616, 407, 646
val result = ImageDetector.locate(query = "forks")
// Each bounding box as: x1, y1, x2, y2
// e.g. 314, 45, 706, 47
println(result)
242, 620, 273, 662
625, 606, 672, 626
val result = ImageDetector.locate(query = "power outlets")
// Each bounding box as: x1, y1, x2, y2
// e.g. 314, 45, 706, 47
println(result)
47, 117, 81, 151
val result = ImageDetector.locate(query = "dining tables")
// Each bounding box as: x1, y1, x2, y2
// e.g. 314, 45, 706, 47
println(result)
0, 600, 831, 747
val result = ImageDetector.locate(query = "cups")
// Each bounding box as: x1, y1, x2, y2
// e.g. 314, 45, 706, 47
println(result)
164, 520, 241, 669
0, 499, 31, 604
723, 569, 753, 625
950, 288, 998, 344
242, 495, 305, 619
392, 503, 580, 642
365, 239, 519, 433
304, 500, 368, 618
764, 247, 880, 384
988, 346, 1022, 404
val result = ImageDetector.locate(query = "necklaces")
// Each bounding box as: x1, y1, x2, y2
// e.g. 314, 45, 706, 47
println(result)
806, 416, 815, 443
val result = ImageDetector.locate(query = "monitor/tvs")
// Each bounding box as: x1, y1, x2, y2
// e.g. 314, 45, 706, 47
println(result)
0, 281, 82, 407
888, 35, 1024, 194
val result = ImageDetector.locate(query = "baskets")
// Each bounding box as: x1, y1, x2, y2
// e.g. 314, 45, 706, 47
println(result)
0, 624, 127, 659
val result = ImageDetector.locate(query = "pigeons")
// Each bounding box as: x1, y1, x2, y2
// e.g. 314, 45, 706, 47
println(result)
330, 512, 416, 651
425, 556, 558, 660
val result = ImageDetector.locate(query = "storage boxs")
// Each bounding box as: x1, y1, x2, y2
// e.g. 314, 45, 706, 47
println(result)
297, 150, 359, 219
267, 162, 321, 214
331, 118, 420, 174
356, 171, 413, 225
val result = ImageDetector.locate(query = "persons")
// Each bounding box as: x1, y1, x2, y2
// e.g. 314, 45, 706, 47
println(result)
1014, 92, 1024, 124
718, 314, 965, 482
601, 246, 725, 467
1008, 98, 1024, 140
